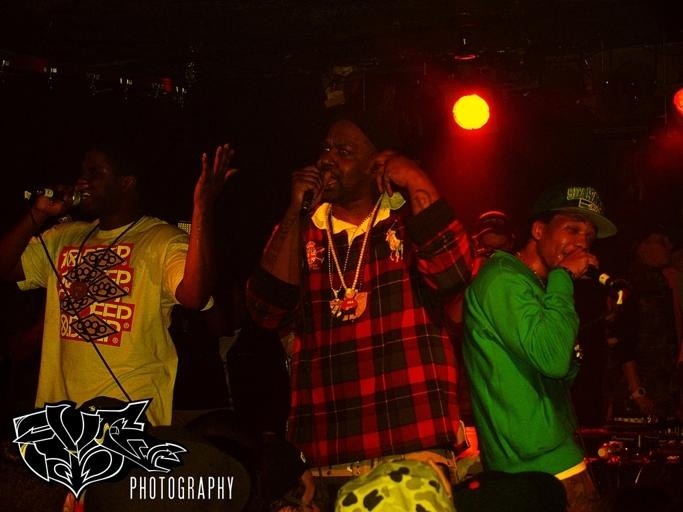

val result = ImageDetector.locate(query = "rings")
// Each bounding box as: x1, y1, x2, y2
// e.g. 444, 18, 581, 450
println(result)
372, 163, 385, 173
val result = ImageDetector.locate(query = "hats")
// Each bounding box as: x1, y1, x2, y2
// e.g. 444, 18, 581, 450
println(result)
525, 181, 618, 241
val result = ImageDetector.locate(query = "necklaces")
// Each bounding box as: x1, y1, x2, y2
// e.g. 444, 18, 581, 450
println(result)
73, 214, 140, 286
324, 192, 390, 324
516, 250, 547, 292
327, 201, 378, 319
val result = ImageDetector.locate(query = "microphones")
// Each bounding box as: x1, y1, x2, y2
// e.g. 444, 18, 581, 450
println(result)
301, 190, 313, 213
585, 265, 617, 288
31, 188, 81, 206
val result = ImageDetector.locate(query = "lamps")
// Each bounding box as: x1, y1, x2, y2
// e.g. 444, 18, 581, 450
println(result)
448, 12, 484, 64
0, 50, 198, 114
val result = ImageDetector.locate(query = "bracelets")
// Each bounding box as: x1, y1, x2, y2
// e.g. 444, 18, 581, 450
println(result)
556, 263, 579, 282
629, 386, 647, 401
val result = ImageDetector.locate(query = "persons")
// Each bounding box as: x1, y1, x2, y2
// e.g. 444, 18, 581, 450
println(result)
576, 246, 613, 422
240, 102, 480, 512
604, 222, 682, 424
462, 201, 519, 275
460, 175, 618, 511
1, 110, 242, 462
